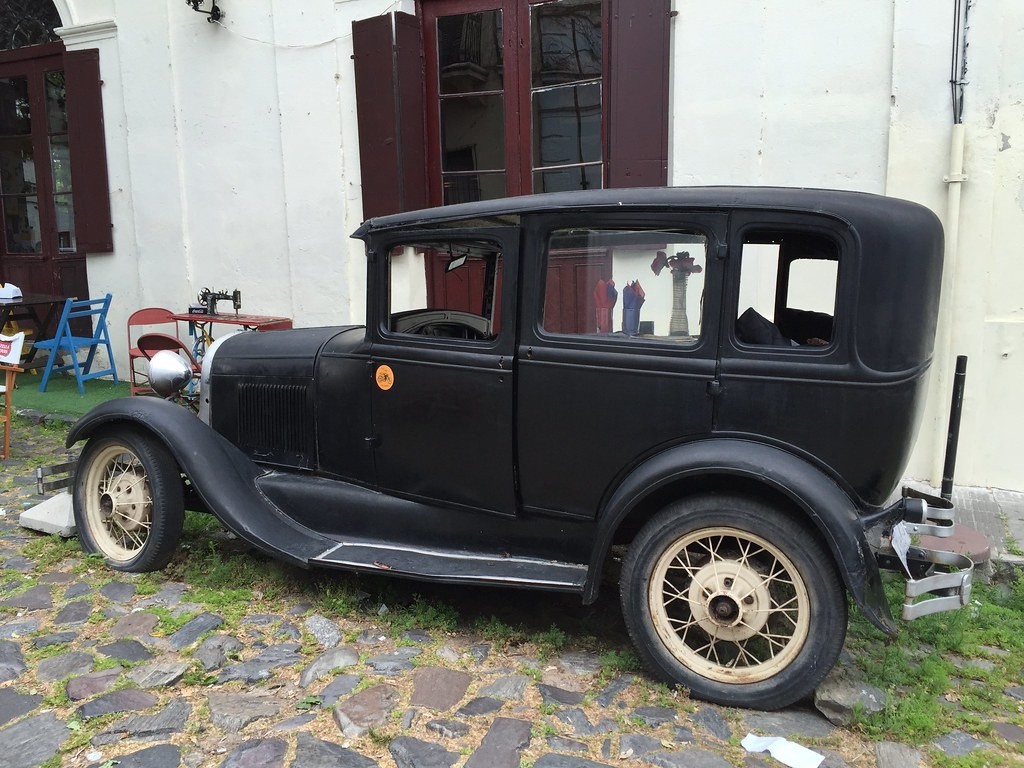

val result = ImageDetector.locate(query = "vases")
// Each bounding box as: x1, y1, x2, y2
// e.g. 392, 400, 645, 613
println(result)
669, 274, 691, 335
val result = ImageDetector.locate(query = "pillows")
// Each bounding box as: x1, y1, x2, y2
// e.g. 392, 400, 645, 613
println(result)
777, 308, 832, 344
735, 308, 790, 347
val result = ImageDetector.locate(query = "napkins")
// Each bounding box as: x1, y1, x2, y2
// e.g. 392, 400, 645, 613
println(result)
620, 279, 647, 332
593, 279, 618, 330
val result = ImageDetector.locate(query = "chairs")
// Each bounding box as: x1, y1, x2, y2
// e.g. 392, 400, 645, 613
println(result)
136, 333, 203, 415
34, 293, 118, 395
128, 308, 179, 398
0, 365, 24, 459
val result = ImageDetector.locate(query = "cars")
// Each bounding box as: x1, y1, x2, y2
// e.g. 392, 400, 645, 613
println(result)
29, 186, 977, 711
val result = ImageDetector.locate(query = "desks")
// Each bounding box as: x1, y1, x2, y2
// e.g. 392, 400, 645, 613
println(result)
0, 292, 78, 379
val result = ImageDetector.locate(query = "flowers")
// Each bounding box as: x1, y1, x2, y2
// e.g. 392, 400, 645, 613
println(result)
650, 248, 702, 277
